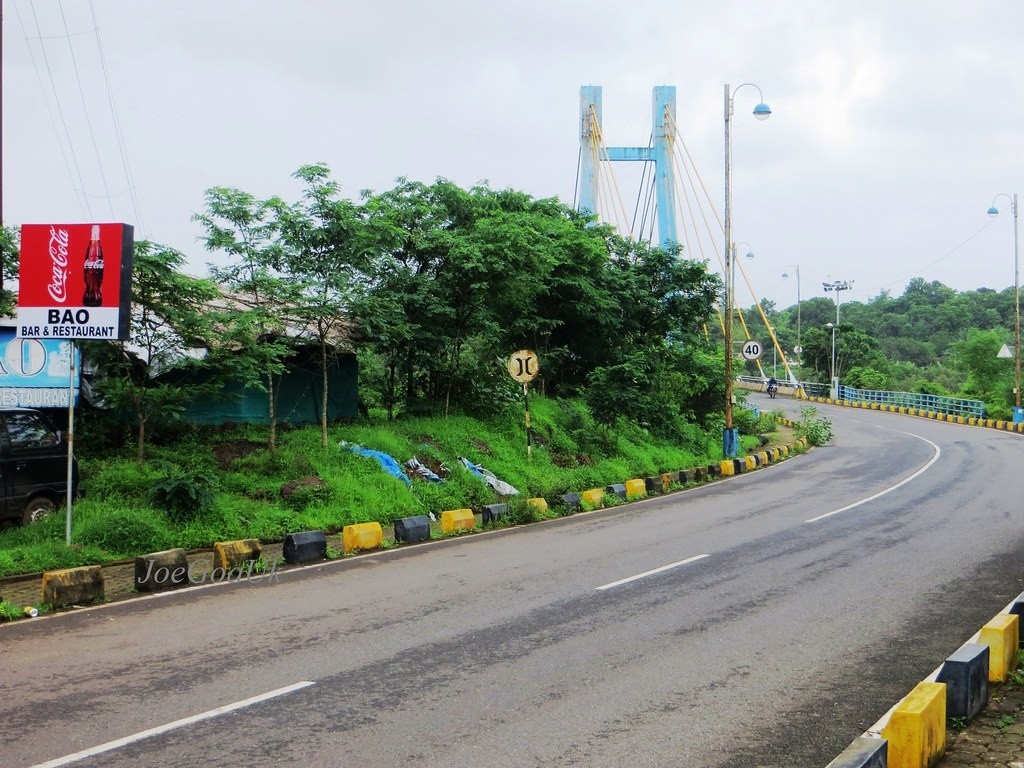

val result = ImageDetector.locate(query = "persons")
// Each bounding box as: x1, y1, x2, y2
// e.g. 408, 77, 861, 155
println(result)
767, 377, 777, 392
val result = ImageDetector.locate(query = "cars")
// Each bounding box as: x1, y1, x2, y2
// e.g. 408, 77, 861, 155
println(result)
0, 408, 78, 525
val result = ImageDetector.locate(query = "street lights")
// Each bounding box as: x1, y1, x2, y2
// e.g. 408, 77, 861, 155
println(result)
822, 278, 854, 325
782, 263, 802, 399
986, 193, 1023, 422
724, 81, 772, 459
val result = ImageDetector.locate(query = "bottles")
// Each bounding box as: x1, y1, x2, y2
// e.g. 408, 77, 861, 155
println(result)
81, 225, 104, 307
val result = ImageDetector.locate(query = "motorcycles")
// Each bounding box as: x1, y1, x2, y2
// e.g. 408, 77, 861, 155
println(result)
768, 382, 777, 397
